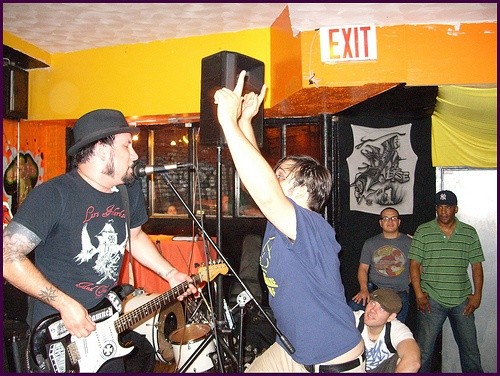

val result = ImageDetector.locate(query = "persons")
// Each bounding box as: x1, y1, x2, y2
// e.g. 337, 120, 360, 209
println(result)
212, 68, 367, 373
351, 287, 422, 373
222, 193, 229, 215
405, 189, 485, 373
346, 207, 415, 324
165, 204, 178, 214
228, 177, 265, 217
3, 108, 199, 373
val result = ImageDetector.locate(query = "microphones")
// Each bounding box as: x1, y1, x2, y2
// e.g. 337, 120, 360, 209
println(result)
132, 162, 192, 178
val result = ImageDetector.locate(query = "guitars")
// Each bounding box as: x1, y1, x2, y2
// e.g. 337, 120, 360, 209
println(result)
24, 258, 229, 373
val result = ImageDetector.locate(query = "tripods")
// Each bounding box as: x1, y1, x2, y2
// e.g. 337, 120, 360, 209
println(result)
178, 146, 240, 374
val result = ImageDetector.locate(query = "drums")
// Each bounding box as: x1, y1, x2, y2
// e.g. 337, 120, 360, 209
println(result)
3, 313, 30, 373
166, 322, 220, 373
121, 287, 185, 367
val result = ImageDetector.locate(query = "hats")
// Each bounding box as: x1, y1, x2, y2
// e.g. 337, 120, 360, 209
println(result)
434, 190, 457, 206
67, 109, 140, 156
369, 289, 402, 314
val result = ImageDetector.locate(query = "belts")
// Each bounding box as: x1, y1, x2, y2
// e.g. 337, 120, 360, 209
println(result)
304, 351, 366, 373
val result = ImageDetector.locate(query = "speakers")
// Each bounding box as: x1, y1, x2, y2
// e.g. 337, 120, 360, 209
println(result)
199, 51, 265, 148
4, 65, 29, 120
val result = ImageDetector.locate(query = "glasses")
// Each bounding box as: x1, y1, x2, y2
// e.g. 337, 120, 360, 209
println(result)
380, 216, 400, 221
